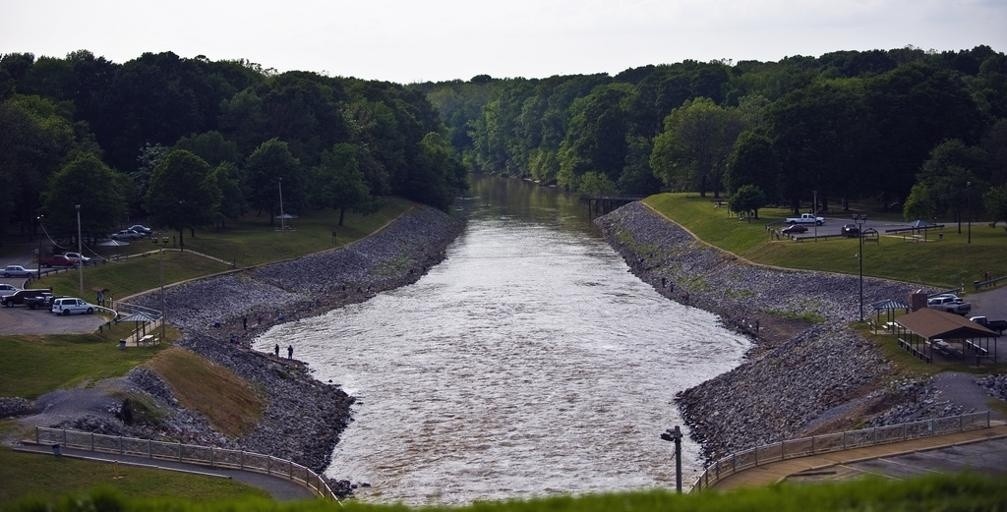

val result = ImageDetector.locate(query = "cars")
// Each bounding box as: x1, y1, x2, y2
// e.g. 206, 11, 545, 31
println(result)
40, 252, 90, 267
0, 284, 93, 315
782, 225, 807, 233
0, 265, 38, 276
842, 223, 858, 237
112, 225, 153, 240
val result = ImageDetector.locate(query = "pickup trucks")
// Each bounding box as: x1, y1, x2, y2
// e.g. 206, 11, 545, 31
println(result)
969, 315, 1006, 336
786, 214, 824, 226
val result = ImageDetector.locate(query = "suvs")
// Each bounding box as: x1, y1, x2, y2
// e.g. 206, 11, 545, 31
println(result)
929, 297, 970, 315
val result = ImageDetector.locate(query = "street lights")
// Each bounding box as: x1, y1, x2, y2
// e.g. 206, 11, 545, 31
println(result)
73, 202, 84, 297
851, 212, 867, 321
179, 200, 184, 246
966, 178, 973, 243
278, 177, 285, 231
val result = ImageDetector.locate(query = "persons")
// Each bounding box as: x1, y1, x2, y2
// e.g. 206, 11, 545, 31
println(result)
275, 343, 279, 359
228, 333, 242, 346
255, 309, 287, 325
214, 319, 219, 328
52, 240, 66, 255
980, 270, 993, 287
242, 315, 248, 331
287, 345, 293, 360
95, 287, 106, 307
